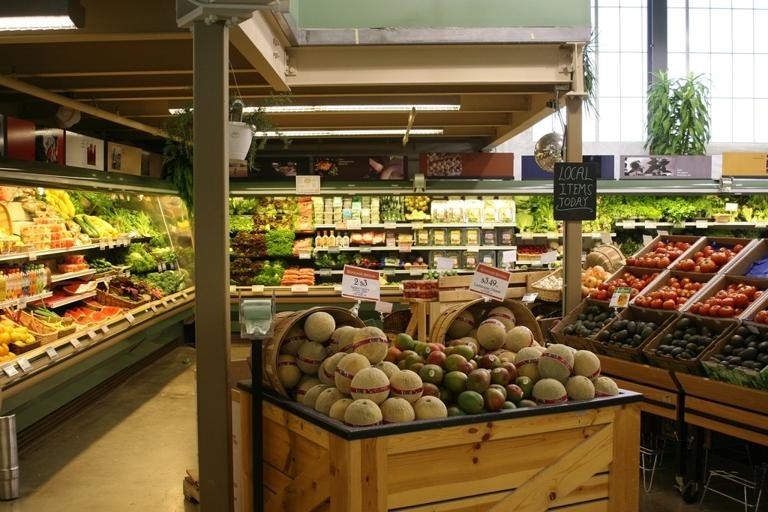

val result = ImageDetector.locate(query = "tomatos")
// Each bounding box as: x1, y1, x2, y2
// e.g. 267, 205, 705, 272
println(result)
591, 240, 768, 323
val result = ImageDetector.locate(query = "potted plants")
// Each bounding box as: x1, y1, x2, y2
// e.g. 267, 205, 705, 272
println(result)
154, 90, 293, 216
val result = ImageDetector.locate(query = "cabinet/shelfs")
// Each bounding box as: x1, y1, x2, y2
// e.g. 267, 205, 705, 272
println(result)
0, 164, 768, 512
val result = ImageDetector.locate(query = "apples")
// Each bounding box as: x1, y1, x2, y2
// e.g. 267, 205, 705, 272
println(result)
581, 265, 605, 298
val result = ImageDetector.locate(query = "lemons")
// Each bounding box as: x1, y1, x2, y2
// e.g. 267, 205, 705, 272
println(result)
0, 320, 36, 363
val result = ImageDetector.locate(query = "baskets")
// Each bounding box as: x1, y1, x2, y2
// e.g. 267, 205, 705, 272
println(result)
0, 306, 76, 354
94, 288, 145, 309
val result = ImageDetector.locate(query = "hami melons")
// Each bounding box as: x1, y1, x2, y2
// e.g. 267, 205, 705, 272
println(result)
277, 306, 618, 428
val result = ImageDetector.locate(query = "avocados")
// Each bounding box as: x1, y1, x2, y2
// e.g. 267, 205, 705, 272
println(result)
562, 305, 768, 372
